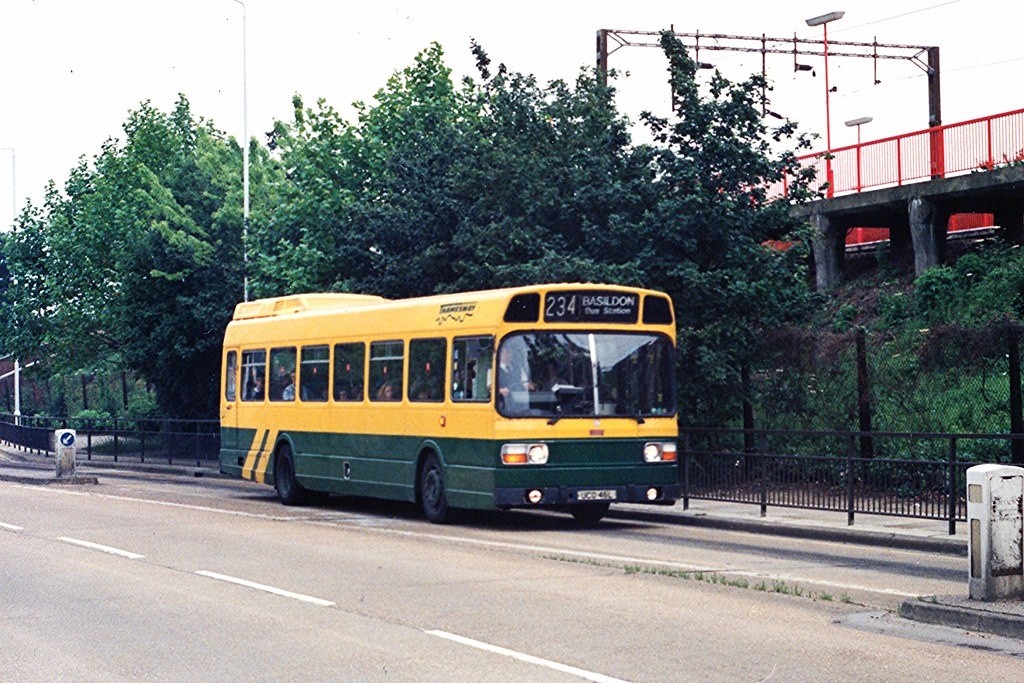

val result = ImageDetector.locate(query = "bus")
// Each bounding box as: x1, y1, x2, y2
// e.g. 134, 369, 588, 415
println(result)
217, 283, 682, 523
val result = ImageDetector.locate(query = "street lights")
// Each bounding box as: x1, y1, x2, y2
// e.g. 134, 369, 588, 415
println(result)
804, 10, 846, 198
844, 115, 873, 193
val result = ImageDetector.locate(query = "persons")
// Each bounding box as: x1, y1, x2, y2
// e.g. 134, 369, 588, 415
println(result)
486, 346, 537, 398
282, 369, 309, 401
378, 382, 401, 400
251, 373, 264, 399
409, 366, 440, 399
